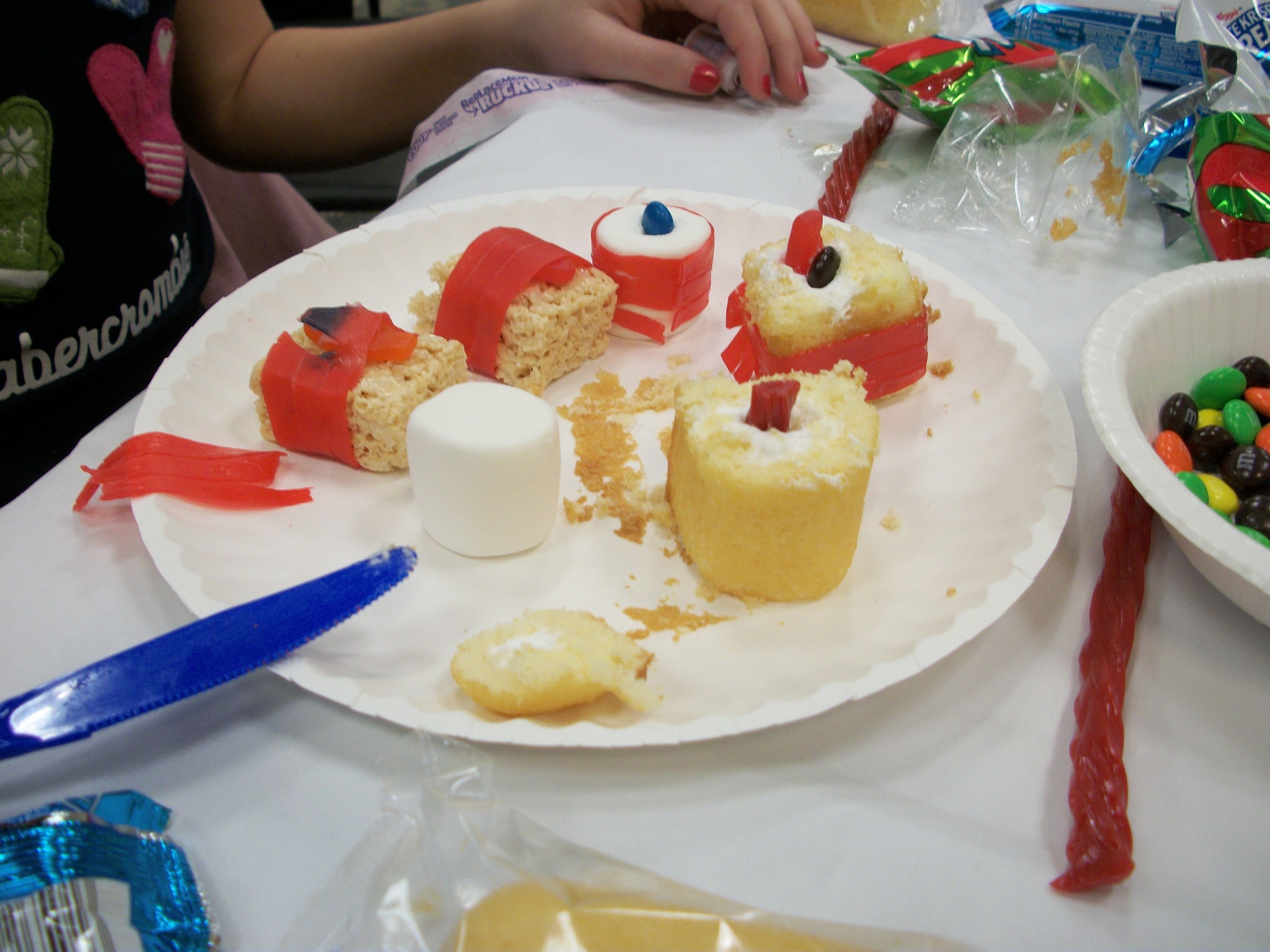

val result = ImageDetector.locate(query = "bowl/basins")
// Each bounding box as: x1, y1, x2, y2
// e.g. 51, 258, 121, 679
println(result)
1082, 256, 1270, 629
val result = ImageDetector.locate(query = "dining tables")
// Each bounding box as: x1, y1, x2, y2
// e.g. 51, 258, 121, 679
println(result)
0, 9, 1270, 952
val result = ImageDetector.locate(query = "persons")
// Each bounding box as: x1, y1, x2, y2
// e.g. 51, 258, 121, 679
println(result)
0, 0, 833, 512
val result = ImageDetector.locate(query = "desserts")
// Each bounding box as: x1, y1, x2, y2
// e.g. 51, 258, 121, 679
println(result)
245, 191, 950, 608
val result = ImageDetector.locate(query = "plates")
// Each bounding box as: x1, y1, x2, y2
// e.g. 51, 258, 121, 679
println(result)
130, 187, 1078, 748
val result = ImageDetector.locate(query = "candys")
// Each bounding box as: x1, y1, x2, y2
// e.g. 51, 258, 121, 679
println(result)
1152, 348, 1269, 553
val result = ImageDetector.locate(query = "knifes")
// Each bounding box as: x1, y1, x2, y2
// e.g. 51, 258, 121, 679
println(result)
0, 547, 418, 762
